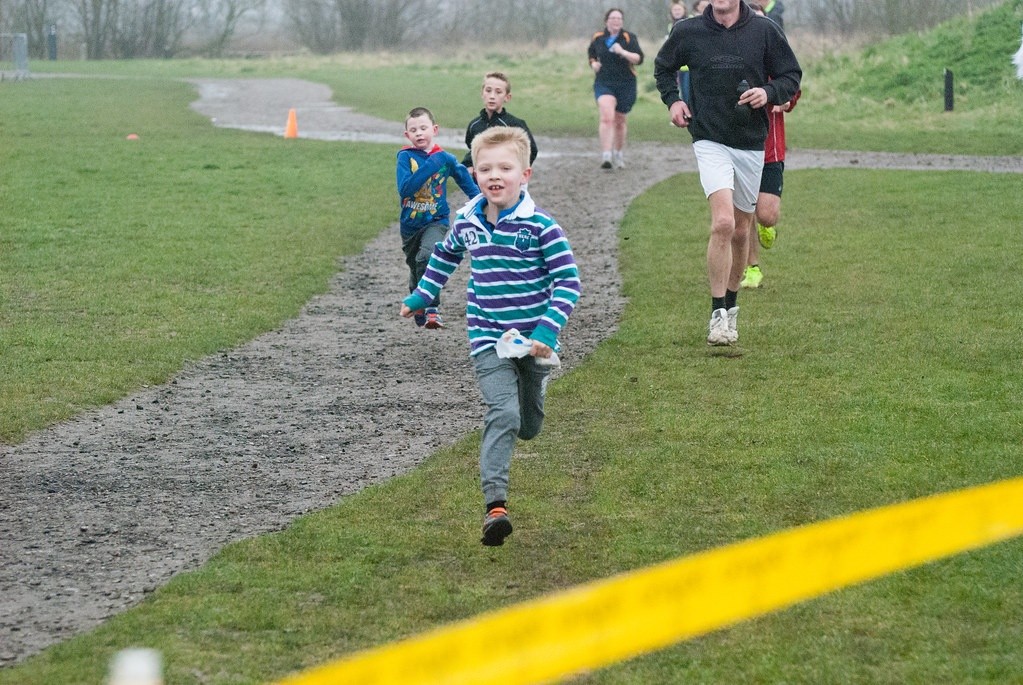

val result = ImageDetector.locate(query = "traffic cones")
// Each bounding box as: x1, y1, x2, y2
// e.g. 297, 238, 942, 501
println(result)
284, 108, 299, 139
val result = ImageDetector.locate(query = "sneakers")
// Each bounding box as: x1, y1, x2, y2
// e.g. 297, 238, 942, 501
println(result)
413, 310, 426, 328
481, 506, 513, 547
615, 158, 624, 169
600, 155, 613, 170
757, 221, 778, 249
741, 264, 763, 289
424, 307, 444, 329
727, 306, 740, 342
706, 308, 731, 345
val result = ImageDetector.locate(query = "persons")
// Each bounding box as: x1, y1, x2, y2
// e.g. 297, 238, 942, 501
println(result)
587, 9, 644, 170
654, 0, 802, 345
399, 125, 581, 549
396, 106, 480, 329
460, 72, 538, 185
664, 0, 802, 289
